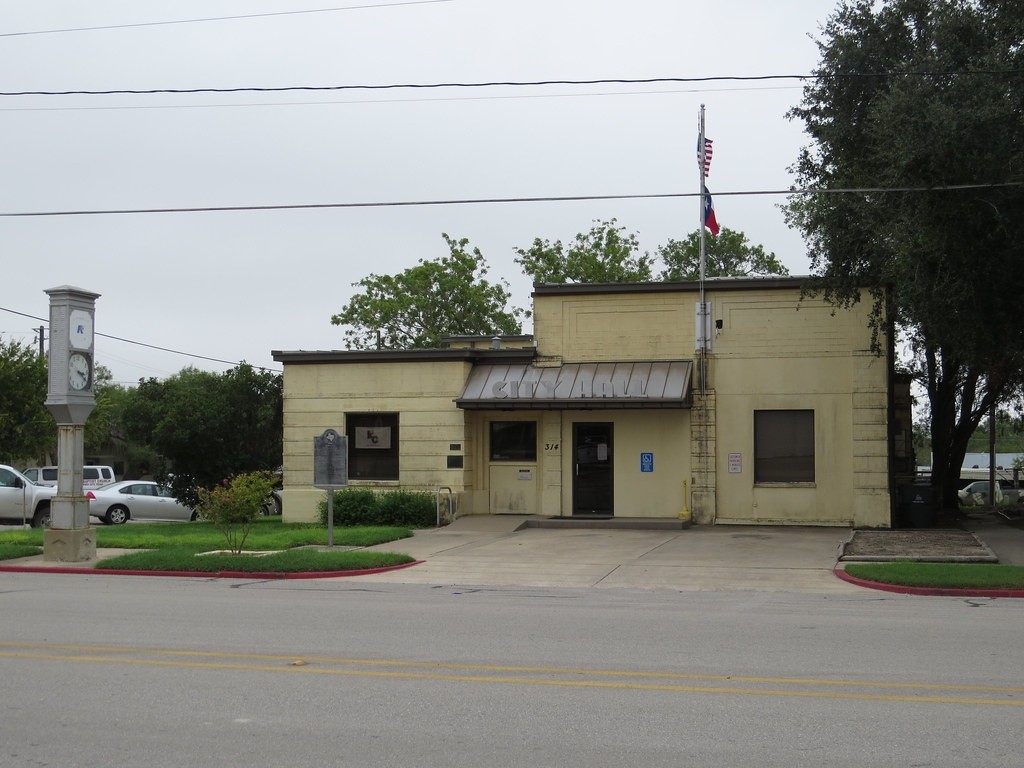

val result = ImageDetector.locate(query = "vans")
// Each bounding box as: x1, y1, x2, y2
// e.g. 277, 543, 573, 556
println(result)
6, 466, 115, 489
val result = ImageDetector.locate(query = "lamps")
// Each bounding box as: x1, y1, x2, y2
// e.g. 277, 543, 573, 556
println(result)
714, 319, 723, 338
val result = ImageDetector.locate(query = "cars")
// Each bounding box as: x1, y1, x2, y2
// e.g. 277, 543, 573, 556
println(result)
84, 480, 199, 525
0, 464, 58, 529
259, 471, 284, 516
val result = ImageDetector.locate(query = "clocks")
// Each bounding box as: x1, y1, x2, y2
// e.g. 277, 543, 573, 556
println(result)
69, 354, 89, 390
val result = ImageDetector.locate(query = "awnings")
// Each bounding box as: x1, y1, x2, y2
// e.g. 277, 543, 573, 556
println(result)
452, 359, 693, 408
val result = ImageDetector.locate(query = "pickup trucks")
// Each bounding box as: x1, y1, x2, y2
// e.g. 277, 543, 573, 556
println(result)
958, 481, 1024, 507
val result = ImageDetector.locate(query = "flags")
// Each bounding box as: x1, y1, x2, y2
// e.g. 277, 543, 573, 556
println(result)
705, 186, 720, 236
697, 134, 713, 178
